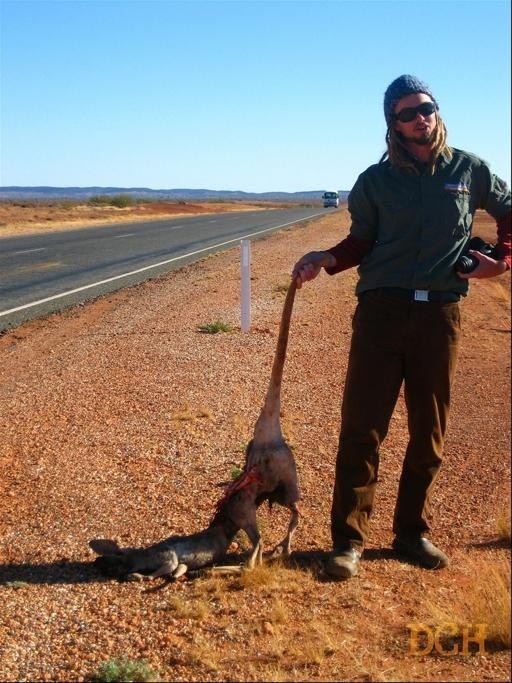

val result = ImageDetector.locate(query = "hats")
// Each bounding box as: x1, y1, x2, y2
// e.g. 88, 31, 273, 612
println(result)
384, 75, 439, 129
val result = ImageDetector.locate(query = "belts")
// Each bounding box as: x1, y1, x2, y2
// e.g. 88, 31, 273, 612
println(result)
369, 286, 459, 303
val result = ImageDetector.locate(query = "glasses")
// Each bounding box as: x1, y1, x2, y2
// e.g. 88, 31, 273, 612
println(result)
394, 101, 436, 122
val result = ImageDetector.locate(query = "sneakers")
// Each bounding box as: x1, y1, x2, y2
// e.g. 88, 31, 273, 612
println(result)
326, 546, 362, 578
392, 535, 449, 569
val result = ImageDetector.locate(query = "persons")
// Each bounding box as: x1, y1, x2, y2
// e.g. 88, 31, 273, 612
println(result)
293, 75, 512, 579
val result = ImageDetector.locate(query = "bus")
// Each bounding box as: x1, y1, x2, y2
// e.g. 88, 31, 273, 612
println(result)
323, 191, 339, 208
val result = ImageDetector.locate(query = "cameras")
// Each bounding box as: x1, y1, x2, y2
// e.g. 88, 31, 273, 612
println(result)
458, 236, 500, 273
